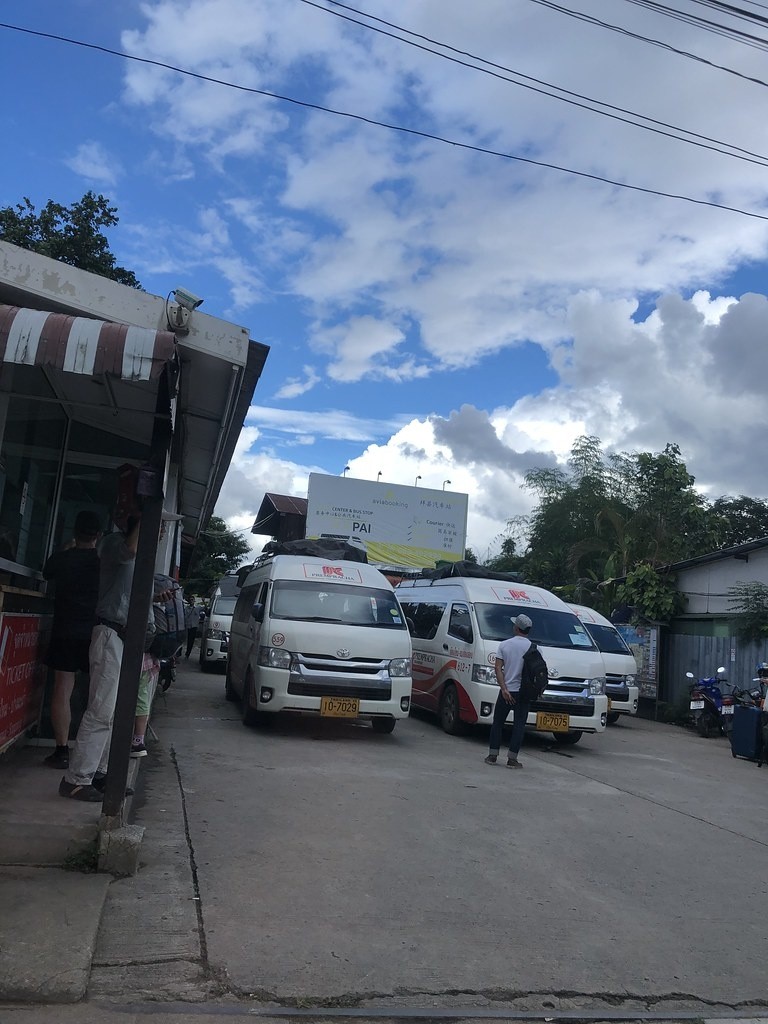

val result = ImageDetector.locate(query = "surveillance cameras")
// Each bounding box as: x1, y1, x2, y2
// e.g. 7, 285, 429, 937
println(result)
173, 287, 204, 310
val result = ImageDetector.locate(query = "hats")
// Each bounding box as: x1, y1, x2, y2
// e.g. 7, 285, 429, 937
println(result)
131, 502, 186, 521
510, 614, 532, 631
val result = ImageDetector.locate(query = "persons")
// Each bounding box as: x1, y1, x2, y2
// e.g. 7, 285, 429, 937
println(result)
184, 596, 207, 660
56, 504, 185, 802
41, 509, 103, 769
129, 651, 160, 757
484, 613, 543, 769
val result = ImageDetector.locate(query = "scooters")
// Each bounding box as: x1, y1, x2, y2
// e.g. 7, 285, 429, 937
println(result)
686, 667, 727, 738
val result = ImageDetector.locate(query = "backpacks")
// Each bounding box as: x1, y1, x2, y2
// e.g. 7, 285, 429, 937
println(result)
518, 643, 548, 700
152, 573, 187, 660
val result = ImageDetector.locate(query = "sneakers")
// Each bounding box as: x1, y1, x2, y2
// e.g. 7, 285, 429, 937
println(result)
130, 743, 147, 757
92, 777, 134, 797
485, 755, 497, 764
507, 758, 522, 768
59, 776, 103, 802
44, 751, 69, 769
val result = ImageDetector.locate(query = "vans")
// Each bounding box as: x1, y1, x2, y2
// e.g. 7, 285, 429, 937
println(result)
566, 602, 638, 723
225, 554, 414, 733
393, 577, 608, 743
200, 570, 238, 672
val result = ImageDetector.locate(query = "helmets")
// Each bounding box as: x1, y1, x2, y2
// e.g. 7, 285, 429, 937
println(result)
756, 662, 768, 674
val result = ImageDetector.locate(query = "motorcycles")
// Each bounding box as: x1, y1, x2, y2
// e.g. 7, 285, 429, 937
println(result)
718, 682, 761, 743
160, 656, 176, 691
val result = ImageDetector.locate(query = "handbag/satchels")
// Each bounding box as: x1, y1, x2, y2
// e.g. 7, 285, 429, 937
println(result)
101, 618, 155, 652
